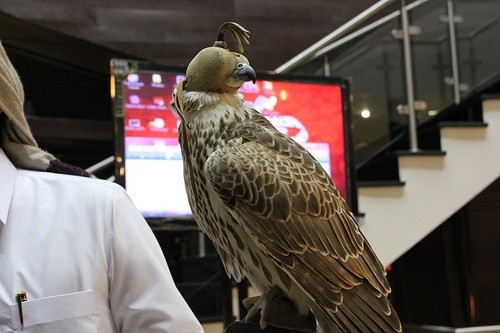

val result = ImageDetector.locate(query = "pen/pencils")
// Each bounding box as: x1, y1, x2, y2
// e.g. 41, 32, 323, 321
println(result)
15, 290, 27, 324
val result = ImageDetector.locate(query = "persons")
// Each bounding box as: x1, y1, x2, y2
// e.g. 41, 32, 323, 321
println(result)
0, 43, 205, 333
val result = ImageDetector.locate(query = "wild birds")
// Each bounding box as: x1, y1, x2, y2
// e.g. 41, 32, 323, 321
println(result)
168, 46, 404, 333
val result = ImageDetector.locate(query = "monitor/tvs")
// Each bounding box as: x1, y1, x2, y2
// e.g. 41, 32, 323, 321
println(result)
111, 59, 359, 230
